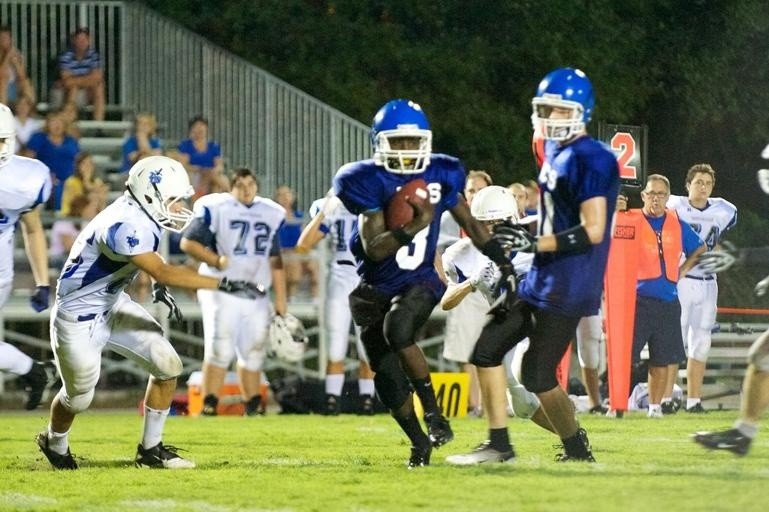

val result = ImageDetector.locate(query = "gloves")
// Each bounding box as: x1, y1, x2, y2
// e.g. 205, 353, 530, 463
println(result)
693, 235, 748, 275
216, 275, 267, 301
469, 261, 493, 288
491, 222, 538, 254
492, 264, 517, 309
150, 282, 183, 324
28, 284, 50, 313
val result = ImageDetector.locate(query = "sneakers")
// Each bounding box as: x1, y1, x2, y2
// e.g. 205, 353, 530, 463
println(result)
133, 441, 196, 471
444, 439, 518, 466
423, 408, 454, 448
693, 426, 750, 456
34, 429, 77, 470
201, 391, 375, 416
21, 359, 61, 411
589, 400, 707, 419
406, 442, 434, 470
554, 425, 596, 463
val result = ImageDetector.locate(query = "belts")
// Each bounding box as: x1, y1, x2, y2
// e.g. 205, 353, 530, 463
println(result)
336, 260, 352, 265
685, 275, 714, 280
77, 309, 108, 322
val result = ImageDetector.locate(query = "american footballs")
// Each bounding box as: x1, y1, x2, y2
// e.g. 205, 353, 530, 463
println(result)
386, 178, 430, 231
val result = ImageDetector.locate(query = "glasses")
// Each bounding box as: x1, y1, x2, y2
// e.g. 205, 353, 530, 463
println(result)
642, 190, 669, 199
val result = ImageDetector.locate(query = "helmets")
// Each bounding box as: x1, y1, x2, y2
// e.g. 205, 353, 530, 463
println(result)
469, 184, 519, 222
125, 154, 198, 227
534, 66, 595, 126
369, 98, 434, 155
0, 103, 17, 140
268, 312, 310, 363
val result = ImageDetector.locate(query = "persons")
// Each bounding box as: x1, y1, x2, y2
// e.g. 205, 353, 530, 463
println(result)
443, 69, 619, 467
335, 102, 519, 466
0, 24, 264, 476
692, 276, 768, 454
180, 163, 738, 416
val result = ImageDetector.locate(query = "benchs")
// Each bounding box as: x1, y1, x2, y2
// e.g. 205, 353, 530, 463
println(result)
6, 97, 194, 297
641, 319, 762, 403
